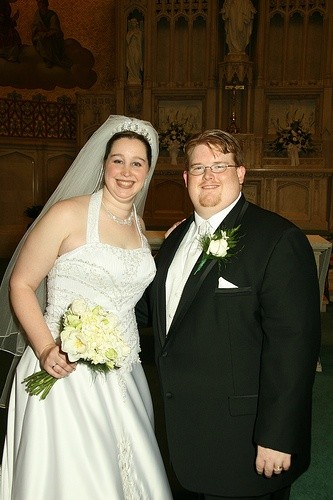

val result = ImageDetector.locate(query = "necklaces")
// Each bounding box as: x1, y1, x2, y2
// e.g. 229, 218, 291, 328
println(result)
100, 201, 134, 226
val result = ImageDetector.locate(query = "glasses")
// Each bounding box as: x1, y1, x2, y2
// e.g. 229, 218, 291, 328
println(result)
187, 162, 238, 176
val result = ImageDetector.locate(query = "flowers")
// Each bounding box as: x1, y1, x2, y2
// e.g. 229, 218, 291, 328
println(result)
21, 299, 130, 398
155, 112, 198, 153
193, 224, 247, 275
269, 110, 320, 154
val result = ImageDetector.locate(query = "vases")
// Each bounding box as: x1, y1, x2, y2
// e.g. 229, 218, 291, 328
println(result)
287, 149, 300, 166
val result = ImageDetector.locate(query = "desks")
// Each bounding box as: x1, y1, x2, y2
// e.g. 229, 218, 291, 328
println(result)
146, 230, 333, 313
144, 165, 333, 239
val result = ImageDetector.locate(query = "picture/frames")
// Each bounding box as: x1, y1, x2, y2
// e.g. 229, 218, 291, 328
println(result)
169, 148, 178, 165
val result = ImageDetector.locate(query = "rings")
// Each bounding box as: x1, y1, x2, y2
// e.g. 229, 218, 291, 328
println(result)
52, 363, 58, 369
273, 466, 283, 471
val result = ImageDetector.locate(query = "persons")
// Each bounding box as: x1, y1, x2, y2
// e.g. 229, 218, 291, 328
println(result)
135, 129, 324, 500
0, 115, 171, 500
125, 19, 144, 84
219, 0, 256, 55
